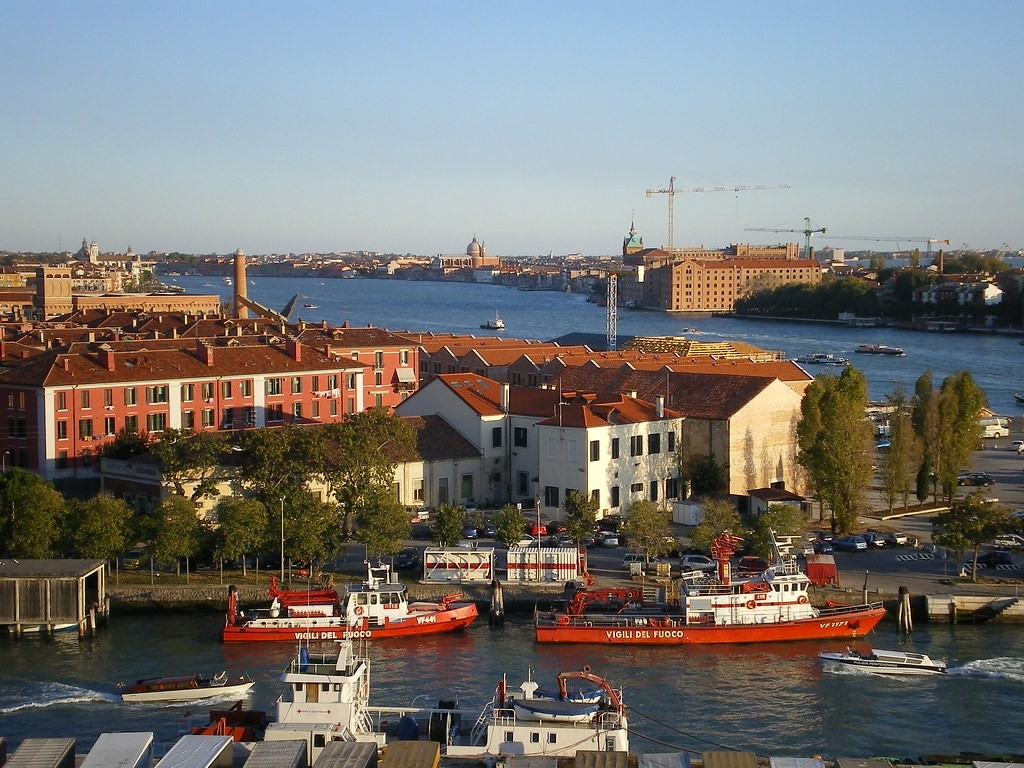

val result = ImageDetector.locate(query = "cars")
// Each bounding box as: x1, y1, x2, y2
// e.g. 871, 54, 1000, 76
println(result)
867, 410, 1024, 485
806, 530, 908, 554
367, 547, 418, 570
679, 554, 716, 574
738, 556, 768, 574
966, 551, 1012, 569
994, 533, 1024, 547
409, 504, 628, 548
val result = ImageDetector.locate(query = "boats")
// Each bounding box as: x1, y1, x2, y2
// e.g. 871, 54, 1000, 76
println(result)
797, 353, 851, 367
854, 344, 906, 358
276, 607, 629, 755
1017, 339, 1024, 347
817, 648, 951, 681
222, 563, 479, 649
163, 271, 322, 311
1013, 393, 1024, 406
535, 528, 887, 646
115, 670, 261, 705
480, 310, 505, 330
886, 594, 1024, 623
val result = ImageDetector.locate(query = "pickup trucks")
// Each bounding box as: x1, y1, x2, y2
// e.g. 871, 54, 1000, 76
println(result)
622, 552, 673, 573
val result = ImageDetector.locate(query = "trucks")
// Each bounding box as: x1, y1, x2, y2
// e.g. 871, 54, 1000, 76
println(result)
264, 722, 357, 768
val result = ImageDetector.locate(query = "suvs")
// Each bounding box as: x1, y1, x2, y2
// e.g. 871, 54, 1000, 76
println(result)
122, 549, 149, 570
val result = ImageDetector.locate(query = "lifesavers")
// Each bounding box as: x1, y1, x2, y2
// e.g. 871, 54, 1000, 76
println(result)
798, 595, 807, 604
354, 606, 364, 616
747, 600, 756, 610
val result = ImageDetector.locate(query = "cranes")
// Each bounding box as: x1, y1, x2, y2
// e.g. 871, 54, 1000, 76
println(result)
743, 216, 828, 259
817, 234, 951, 267
645, 176, 792, 250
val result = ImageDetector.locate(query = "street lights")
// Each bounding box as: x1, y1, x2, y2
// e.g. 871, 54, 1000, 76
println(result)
536, 499, 541, 584
280, 494, 287, 584
